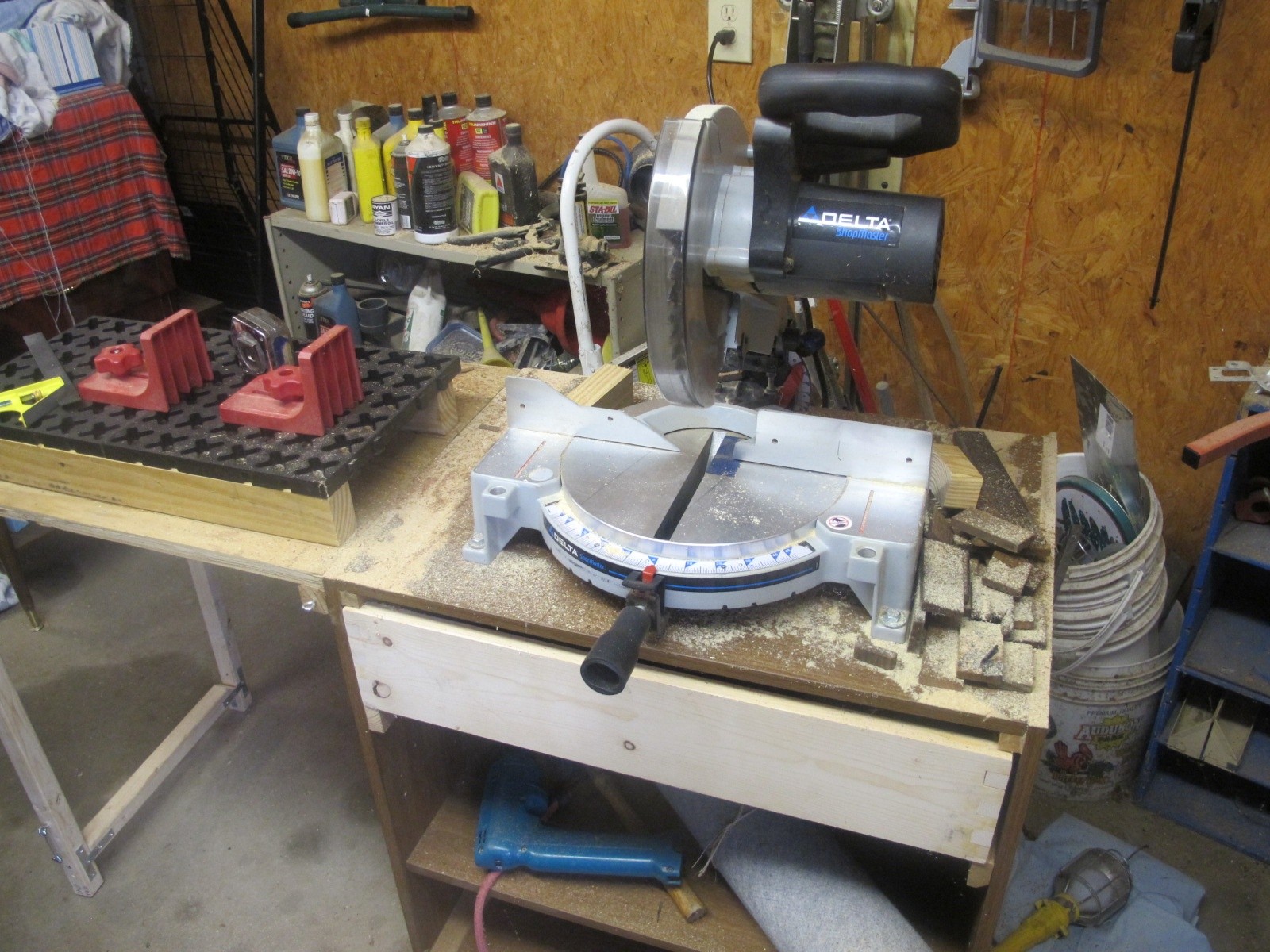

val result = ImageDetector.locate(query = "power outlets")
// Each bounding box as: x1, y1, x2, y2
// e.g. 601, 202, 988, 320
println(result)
707, 0, 752, 64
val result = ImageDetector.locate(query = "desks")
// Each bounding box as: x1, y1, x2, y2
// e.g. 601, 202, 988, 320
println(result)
0, 362, 1058, 952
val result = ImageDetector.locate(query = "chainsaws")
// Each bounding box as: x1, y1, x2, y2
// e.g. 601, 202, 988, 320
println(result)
644, 53, 966, 410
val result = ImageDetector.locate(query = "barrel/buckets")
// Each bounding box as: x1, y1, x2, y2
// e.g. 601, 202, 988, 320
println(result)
1030, 450, 1188, 798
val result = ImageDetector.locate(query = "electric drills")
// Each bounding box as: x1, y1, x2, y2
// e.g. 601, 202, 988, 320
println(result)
474, 741, 685, 888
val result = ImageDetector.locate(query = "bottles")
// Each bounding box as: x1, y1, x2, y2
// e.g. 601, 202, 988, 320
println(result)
297, 112, 350, 223
405, 123, 462, 246
351, 117, 388, 224
298, 273, 328, 340
371, 103, 405, 144
382, 91, 474, 205
401, 258, 448, 355
272, 106, 310, 210
330, 191, 359, 225
313, 273, 363, 347
487, 122, 541, 228
577, 133, 632, 250
465, 95, 512, 194
451, 171, 501, 234
556, 176, 592, 244
332, 112, 361, 215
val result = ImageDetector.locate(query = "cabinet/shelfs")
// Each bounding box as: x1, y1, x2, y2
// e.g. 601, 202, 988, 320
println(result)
1132, 367, 1270, 858
266, 204, 644, 385
